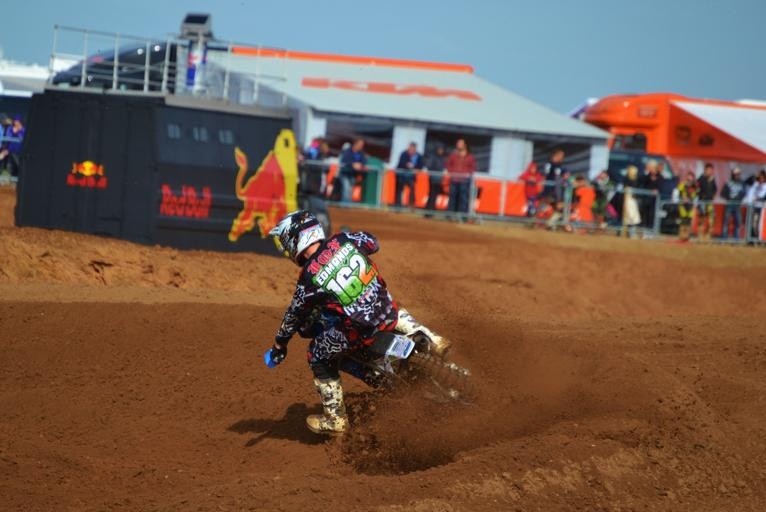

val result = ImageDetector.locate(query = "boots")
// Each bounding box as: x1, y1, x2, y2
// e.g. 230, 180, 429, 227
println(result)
307, 381, 350, 437
395, 307, 452, 357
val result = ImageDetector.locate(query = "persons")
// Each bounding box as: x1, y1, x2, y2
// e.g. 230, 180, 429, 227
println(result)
297, 139, 367, 199
520, 149, 682, 240
395, 143, 424, 210
0, 115, 12, 171
265, 209, 451, 439
721, 167, 766, 240
7, 114, 25, 175
424, 139, 476, 222
679, 163, 717, 242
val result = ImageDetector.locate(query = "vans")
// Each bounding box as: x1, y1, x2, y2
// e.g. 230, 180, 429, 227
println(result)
608, 149, 680, 233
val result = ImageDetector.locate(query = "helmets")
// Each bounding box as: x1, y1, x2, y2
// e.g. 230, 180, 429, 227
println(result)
268, 210, 325, 265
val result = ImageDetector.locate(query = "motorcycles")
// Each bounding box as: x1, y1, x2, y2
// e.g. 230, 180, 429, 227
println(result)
273, 304, 484, 418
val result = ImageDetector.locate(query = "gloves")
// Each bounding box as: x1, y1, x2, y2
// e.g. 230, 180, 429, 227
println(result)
270, 345, 288, 365
